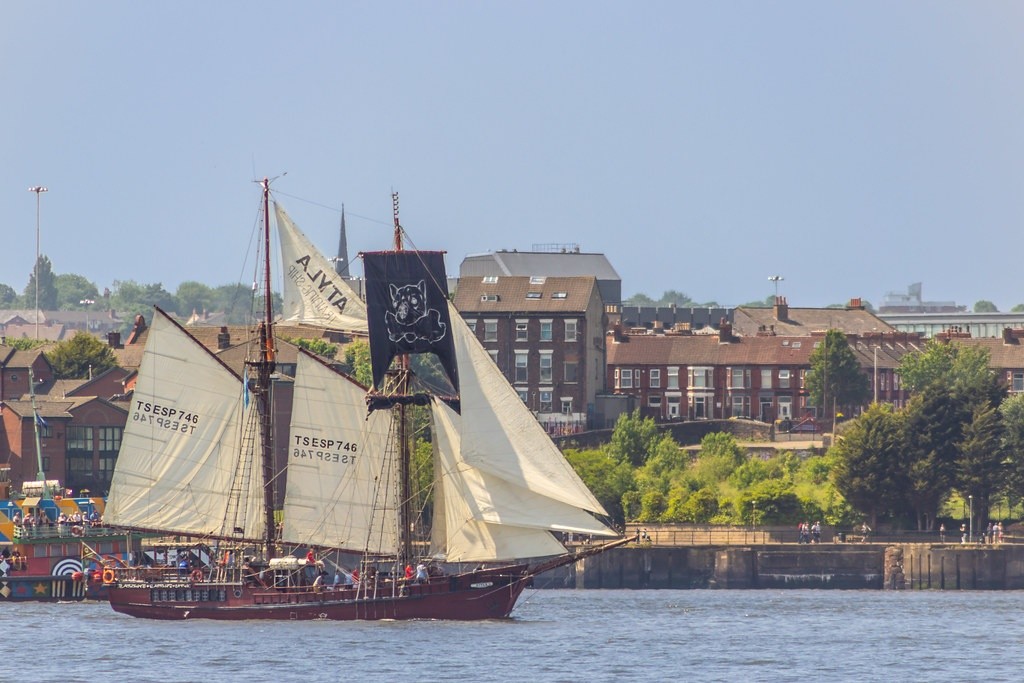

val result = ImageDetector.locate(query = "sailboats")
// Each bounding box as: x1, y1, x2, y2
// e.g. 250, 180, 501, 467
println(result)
88, 175, 642, 622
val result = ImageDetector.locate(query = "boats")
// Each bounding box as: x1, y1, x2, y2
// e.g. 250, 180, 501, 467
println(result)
0, 496, 149, 603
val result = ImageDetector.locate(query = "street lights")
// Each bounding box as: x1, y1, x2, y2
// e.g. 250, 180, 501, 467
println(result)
28, 186, 48, 342
80, 299, 95, 333
752, 500, 756, 543
768, 276, 784, 304
874, 346, 881, 402
968, 495, 972, 542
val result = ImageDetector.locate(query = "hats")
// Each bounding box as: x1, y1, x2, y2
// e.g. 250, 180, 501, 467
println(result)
321, 571, 328, 575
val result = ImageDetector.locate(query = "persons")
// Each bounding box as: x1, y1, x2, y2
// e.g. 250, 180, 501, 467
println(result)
861, 522, 871, 542
179, 558, 193, 576
987, 522, 1003, 544
642, 528, 652, 542
306, 549, 428, 593
2, 546, 21, 558
13, 510, 100, 538
940, 523, 945, 543
798, 520, 821, 544
960, 524, 968, 543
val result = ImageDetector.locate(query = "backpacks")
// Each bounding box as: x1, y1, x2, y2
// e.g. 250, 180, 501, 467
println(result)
799, 523, 806, 532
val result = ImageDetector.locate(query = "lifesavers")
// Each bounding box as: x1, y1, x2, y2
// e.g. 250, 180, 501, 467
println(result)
190, 569, 203, 583
102, 569, 114, 583
